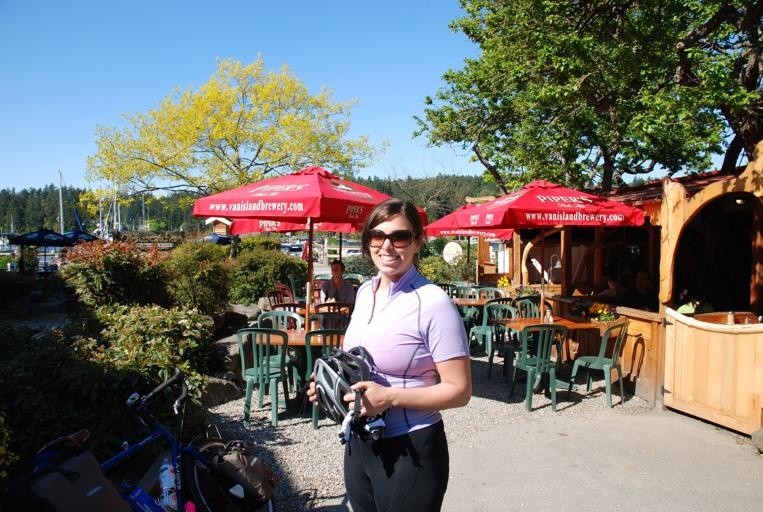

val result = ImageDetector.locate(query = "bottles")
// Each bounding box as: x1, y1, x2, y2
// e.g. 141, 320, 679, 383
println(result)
544, 309, 553, 323
311, 318, 319, 331
727, 311, 735, 325
159, 458, 177, 510
117, 480, 166, 512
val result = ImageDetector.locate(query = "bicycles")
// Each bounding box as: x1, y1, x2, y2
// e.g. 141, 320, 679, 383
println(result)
31, 366, 274, 512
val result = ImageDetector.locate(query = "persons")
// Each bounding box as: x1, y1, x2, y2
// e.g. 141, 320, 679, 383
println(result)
594, 279, 620, 298
619, 268, 659, 314
8, 252, 22, 274
676, 288, 714, 314
306, 197, 472, 511
318, 258, 357, 308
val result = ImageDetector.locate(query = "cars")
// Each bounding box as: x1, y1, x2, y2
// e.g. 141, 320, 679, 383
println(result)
203, 232, 362, 275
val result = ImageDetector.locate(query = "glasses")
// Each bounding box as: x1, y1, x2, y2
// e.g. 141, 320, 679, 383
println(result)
366, 229, 416, 248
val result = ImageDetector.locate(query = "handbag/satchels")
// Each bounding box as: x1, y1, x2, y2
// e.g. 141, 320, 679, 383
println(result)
218, 438, 276, 507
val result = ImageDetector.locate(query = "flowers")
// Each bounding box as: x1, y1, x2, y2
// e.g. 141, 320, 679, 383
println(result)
515, 285, 540, 299
597, 304, 619, 322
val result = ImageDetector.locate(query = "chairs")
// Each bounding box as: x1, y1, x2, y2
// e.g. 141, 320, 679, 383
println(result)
236, 328, 287, 428
256, 311, 305, 394
486, 304, 535, 383
568, 321, 631, 409
310, 313, 349, 372
305, 329, 346, 430
0, 261, 81, 323
267, 273, 554, 357
517, 300, 562, 371
508, 324, 568, 413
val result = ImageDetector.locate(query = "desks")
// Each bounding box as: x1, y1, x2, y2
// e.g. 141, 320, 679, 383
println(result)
489, 315, 598, 390
256, 330, 344, 417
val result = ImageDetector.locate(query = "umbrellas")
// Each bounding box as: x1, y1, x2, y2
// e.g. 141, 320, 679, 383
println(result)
8, 225, 70, 270
219, 219, 368, 258
453, 177, 646, 326
192, 163, 428, 335
63, 228, 101, 246
421, 188, 519, 300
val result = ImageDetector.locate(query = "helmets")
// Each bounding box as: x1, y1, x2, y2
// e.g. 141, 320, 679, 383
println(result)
314, 345, 392, 441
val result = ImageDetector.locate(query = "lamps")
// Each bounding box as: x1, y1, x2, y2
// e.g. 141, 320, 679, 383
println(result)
547, 254, 562, 285
